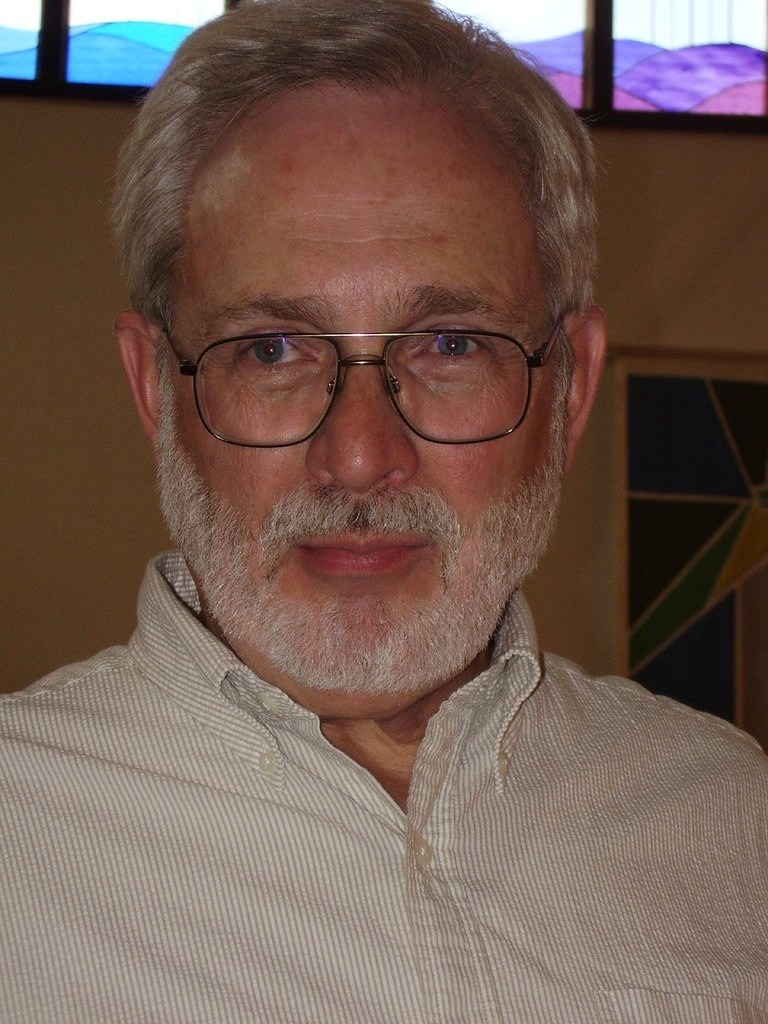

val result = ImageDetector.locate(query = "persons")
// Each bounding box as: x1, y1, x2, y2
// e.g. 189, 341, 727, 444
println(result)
0, 0, 768, 1024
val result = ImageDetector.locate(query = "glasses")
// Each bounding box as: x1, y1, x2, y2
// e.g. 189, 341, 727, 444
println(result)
162, 307, 565, 448
